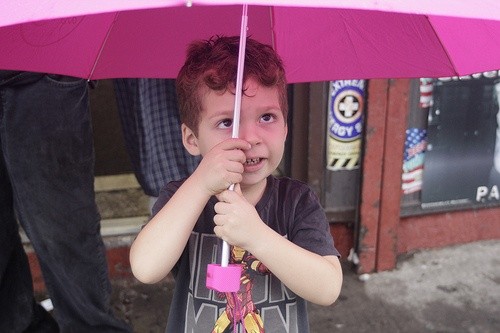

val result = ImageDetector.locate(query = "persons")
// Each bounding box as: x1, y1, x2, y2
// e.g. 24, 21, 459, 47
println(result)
0, 70, 134, 333
129, 35, 343, 333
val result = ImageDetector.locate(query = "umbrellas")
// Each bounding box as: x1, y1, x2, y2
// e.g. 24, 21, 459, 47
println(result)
1, 0, 500, 82
